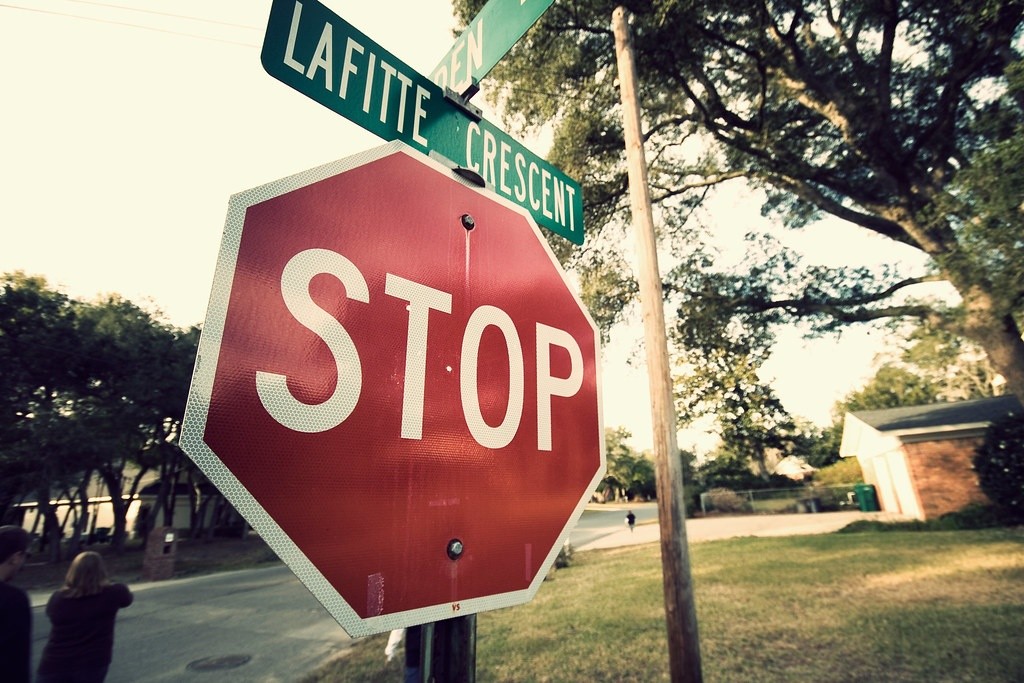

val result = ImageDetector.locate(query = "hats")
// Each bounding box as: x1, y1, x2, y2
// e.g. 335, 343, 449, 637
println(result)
0, 525, 28, 566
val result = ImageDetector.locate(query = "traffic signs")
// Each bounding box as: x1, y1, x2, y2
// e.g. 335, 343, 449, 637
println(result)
260, 0, 587, 244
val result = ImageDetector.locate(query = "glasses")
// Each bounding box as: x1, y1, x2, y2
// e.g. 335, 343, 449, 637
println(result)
20, 551, 32, 560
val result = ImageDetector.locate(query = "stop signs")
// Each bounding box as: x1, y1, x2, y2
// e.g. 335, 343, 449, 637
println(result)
180, 139, 607, 638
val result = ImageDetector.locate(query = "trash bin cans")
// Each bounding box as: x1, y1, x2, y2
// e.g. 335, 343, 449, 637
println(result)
854, 484, 880, 512
140, 527, 179, 579
797, 496, 821, 514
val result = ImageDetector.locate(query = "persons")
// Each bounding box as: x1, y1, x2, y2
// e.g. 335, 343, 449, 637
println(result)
36, 552, 133, 683
0, 525, 34, 683
627, 511, 635, 531
